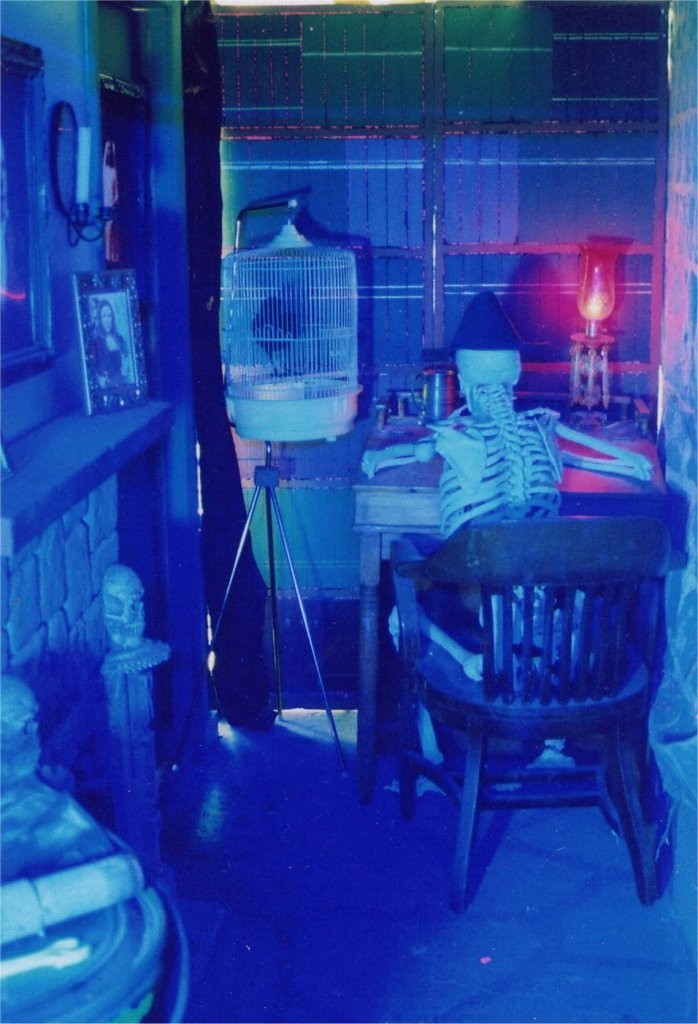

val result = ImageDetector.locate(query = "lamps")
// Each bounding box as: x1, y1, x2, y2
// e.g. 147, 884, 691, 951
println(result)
567, 231, 619, 408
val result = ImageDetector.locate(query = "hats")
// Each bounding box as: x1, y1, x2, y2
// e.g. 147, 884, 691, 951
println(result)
448, 290, 522, 357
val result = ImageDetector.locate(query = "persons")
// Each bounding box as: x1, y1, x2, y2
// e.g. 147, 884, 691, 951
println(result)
92, 298, 129, 387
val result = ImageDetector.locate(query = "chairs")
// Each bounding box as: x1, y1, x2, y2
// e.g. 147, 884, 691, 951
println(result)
388, 514, 676, 922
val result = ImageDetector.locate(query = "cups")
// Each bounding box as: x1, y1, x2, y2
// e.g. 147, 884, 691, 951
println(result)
413, 370, 455, 426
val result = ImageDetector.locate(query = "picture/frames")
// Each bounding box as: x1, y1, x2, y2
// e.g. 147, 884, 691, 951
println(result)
0, 35, 62, 389
68, 263, 153, 418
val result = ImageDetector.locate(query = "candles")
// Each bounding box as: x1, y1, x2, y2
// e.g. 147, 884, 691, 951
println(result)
77, 126, 91, 204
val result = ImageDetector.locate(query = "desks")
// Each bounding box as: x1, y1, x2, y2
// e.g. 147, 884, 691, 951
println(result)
350, 444, 692, 811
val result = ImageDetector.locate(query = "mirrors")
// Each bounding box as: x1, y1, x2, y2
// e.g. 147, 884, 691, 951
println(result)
49, 99, 79, 217
